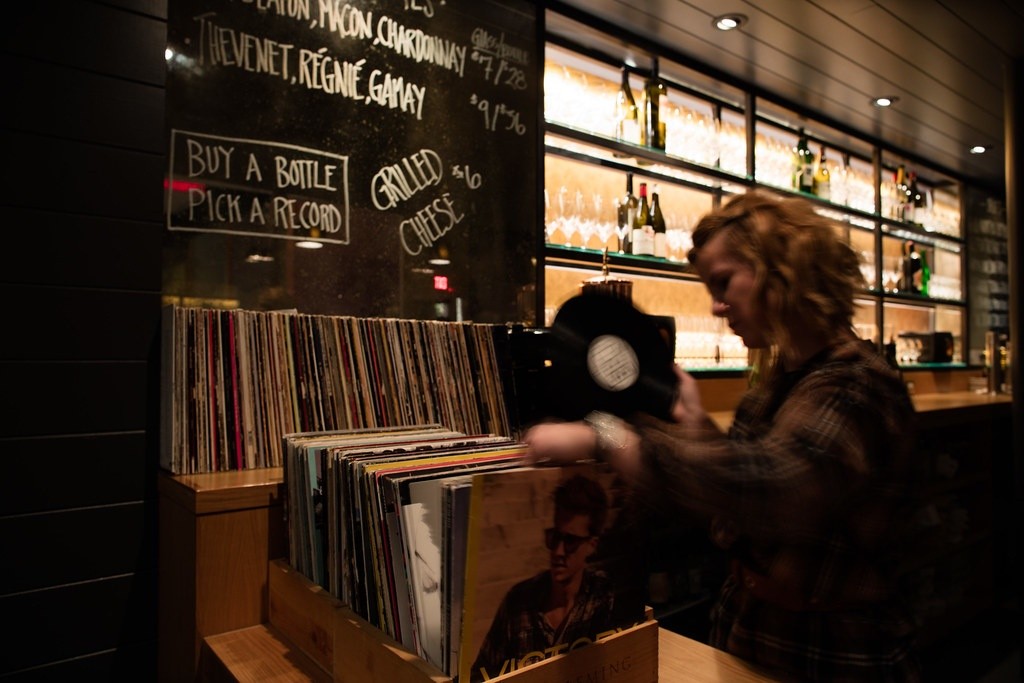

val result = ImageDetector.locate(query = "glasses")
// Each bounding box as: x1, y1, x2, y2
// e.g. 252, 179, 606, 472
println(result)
543, 525, 593, 555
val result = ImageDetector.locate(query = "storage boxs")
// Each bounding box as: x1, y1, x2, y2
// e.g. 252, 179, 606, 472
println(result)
268, 557, 659, 683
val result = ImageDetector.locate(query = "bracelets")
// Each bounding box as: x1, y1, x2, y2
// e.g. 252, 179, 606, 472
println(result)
584, 410, 619, 462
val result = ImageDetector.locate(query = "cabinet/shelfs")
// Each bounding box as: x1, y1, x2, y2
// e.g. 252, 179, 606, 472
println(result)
541, 1, 1014, 379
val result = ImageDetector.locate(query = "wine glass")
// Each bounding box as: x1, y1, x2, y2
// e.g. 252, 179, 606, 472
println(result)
544, 61, 628, 145
664, 212, 695, 264
577, 194, 599, 249
665, 102, 806, 191
615, 197, 632, 255
895, 339, 922, 365
595, 197, 618, 253
858, 251, 904, 292
674, 317, 748, 368
545, 189, 563, 244
815, 158, 962, 238
555, 188, 580, 246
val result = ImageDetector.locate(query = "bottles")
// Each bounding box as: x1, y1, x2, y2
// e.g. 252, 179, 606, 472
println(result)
793, 126, 830, 198
637, 57, 667, 166
903, 240, 921, 296
890, 166, 923, 228
613, 66, 638, 158
631, 183, 654, 257
650, 184, 666, 259
619, 171, 638, 254
919, 249, 930, 297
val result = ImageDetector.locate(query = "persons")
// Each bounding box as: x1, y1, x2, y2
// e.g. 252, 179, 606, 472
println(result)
707, 515, 742, 549
470, 476, 645, 683
521, 192, 930, 683
